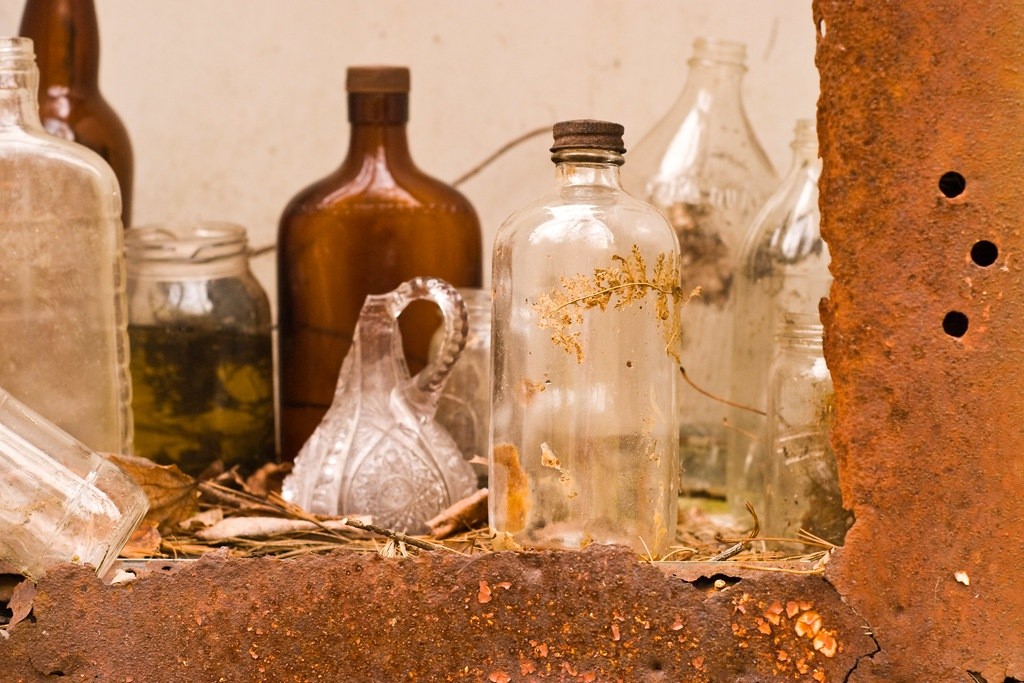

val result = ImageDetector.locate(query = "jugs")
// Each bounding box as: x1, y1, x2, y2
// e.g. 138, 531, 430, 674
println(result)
281, 274, 479, 536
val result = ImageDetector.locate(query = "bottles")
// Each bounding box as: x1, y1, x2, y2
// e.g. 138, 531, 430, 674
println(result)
725, 117, 850, 561
492, 121, 685, 551
119, 221, 274, 492
277, 66, 483, 457
0, 35, 134, 457
0, 381, 149, 579
19, 1, 134, 221
426, 288, 490, 461
604, 35, 781, 539
